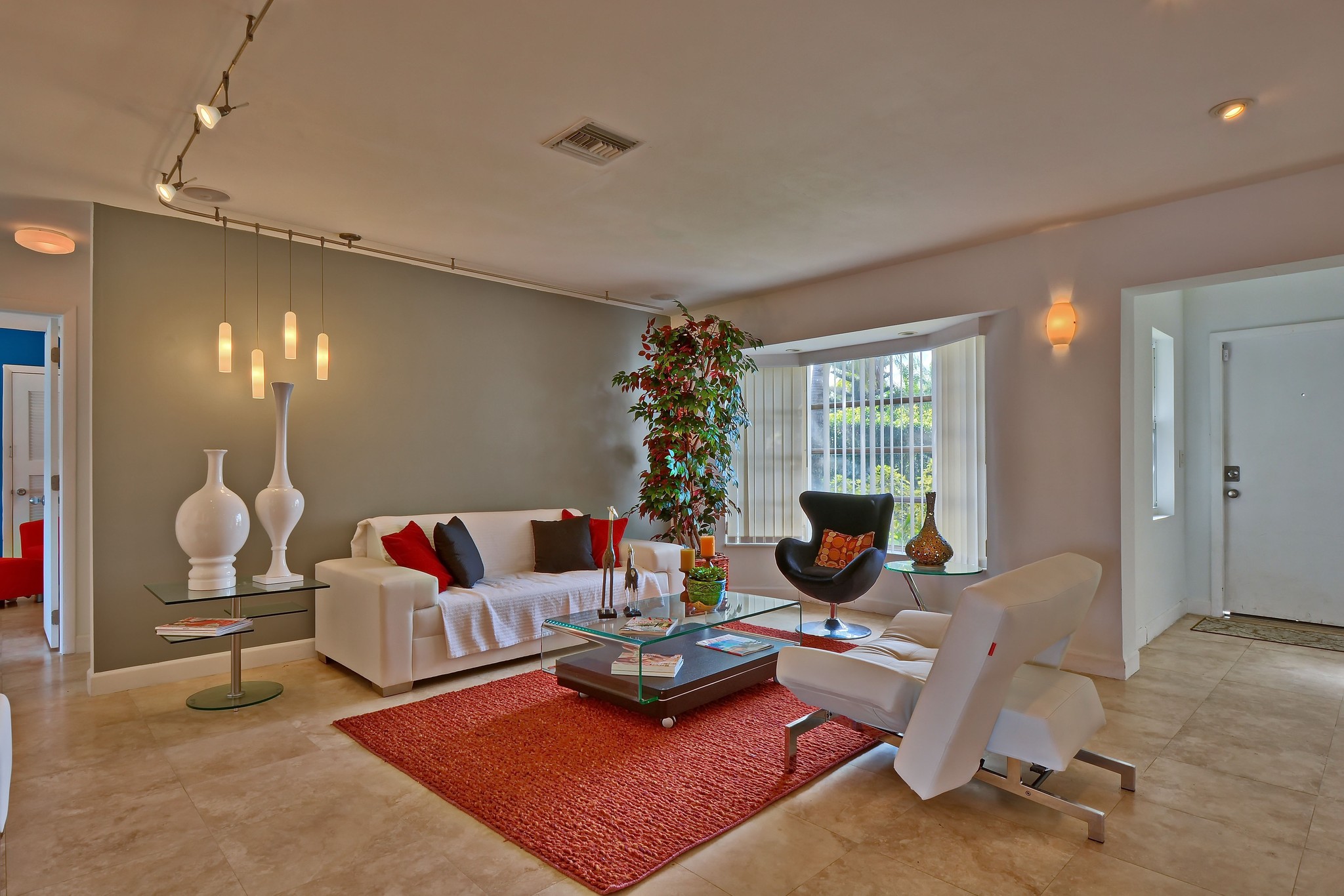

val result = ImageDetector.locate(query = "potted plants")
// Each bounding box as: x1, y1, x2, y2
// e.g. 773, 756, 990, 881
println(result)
612, 297, 763, 606
685, 566, 727, 612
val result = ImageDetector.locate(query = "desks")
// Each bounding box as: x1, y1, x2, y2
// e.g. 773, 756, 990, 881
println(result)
883, 558, 985, 610
142, 570, 334, 712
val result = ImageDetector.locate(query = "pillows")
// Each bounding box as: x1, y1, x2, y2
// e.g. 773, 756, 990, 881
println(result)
527, 514, 599, 575
816, 529, 876, 570
559, 506, 628, 569
379, 520, 455, 595
432, 517, 484, 589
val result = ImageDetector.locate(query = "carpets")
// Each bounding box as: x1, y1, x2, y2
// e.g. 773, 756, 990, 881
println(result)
1188, 613, 1342, 653
333, 622, 901, 896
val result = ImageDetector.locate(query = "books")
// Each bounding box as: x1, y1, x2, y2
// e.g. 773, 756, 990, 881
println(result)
611, 652, 684, 678
619, 616, 679, 636
155, 617, 253, 636
696, 634, 774, 656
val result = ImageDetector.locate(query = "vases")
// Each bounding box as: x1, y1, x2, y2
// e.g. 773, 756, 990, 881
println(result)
170, 447, 251, 594
907, 491, 954, 566
252, 378, 308, 586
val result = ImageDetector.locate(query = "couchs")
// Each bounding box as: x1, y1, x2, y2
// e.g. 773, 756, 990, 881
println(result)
313, 508, 689, 699
773, 549, 1138, 844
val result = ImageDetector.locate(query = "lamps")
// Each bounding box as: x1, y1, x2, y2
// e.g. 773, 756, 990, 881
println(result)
215, 220, 335, 404
12, 227, 77, 257
153, 69, 245, 208
1044, 302, 1085, 348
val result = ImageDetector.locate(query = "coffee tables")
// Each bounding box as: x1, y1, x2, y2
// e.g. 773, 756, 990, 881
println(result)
536, 589, 804, 729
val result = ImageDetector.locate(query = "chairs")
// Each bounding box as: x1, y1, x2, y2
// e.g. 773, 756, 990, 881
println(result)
0, 520, 45, 607
770, 490, 895, 641
